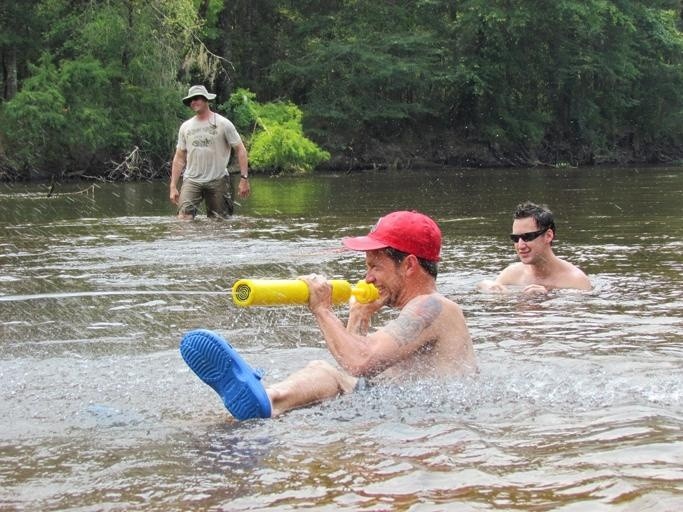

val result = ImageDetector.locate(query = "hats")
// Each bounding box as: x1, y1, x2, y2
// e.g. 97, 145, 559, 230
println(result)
181, 85, 216, 105
342, 211, 441, 263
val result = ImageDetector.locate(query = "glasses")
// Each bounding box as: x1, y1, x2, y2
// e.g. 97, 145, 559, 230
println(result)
510, 230, 549, 242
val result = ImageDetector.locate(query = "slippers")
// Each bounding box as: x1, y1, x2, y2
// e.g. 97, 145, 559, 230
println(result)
181, 330, 270, 420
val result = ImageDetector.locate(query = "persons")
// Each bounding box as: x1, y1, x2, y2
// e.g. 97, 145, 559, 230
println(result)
170, 84, 251, 220
180, 211, 477, 421
486, 201, 592, 294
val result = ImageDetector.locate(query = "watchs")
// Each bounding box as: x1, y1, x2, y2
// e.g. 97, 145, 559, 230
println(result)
241, 175, 249, 179
